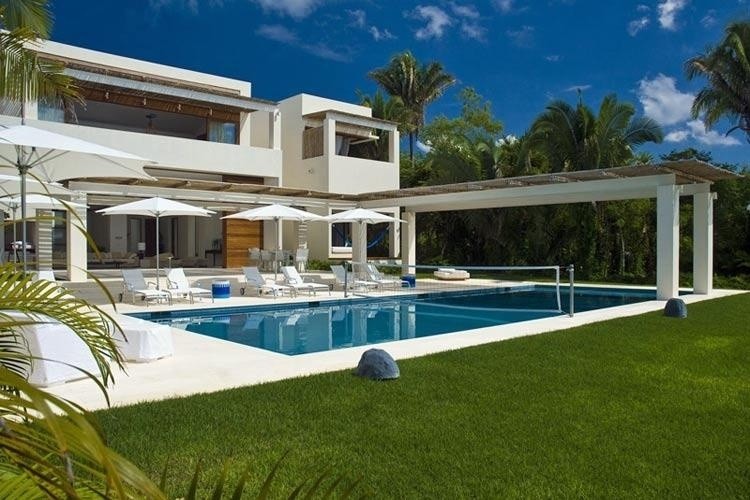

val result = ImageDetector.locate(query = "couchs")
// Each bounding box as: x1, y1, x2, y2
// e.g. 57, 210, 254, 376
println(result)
87, 251, 139, 268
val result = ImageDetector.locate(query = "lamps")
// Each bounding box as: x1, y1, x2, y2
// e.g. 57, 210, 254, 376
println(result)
138, 242, 146, 259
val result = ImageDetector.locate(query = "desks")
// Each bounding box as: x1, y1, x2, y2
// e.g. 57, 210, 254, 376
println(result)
212, 280, 230, 298
205, 250, 223, 266
403, 274, 416, 288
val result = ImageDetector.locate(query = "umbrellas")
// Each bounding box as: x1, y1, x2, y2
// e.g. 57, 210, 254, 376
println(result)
0, 174, 79, 200
0, 193, 89, 288
95, 194, 217, 291
323, 205, 408, 279
219, 202, 322, 281
0, 125, 158, 296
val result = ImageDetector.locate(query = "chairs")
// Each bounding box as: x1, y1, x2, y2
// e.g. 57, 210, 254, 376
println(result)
248, 246, 291, 273
330, 262, 412, 295
121, 268, 214, 306
240, 265, 331, 298
8, 240, 35, 263
